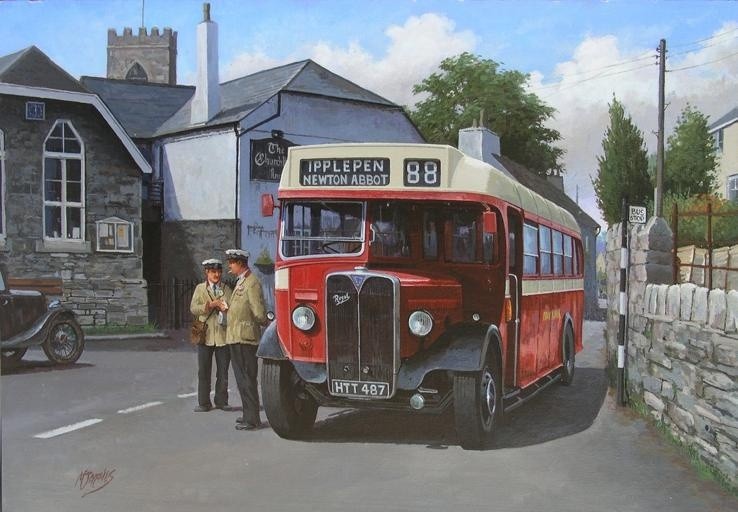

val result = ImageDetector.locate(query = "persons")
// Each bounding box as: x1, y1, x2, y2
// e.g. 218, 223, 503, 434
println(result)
224, 248, 272, 431
189, 257, 233, 412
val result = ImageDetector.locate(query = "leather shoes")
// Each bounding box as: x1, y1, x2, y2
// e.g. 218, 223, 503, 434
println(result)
236, 416, 260, 430
194, 405, 212, 412
216, 404, 233, 411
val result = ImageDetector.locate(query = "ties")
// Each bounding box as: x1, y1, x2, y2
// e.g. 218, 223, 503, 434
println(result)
213, 284, 216, 297
233, 277, 240, 293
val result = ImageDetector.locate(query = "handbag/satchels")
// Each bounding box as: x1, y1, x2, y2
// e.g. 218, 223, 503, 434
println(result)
188, 319, 208, 346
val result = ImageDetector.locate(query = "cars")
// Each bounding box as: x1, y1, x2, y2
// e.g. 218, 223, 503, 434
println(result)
0, 265, 85, 367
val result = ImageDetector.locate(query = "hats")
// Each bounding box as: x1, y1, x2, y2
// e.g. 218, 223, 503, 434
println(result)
202, 258, 223, 269
225, 249, 250, 260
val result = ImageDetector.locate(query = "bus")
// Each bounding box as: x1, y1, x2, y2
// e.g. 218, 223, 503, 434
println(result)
255, 143, 585, 450
